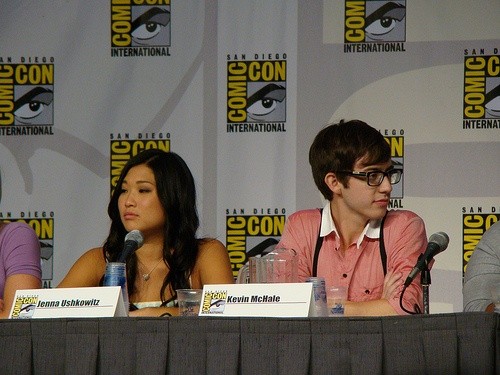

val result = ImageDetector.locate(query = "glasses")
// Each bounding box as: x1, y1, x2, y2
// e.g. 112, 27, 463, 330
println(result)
331, 169, 403, 186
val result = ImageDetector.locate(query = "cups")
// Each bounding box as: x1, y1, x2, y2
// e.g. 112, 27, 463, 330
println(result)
103, 262, 130, 317
304, 276, 328, 318
176, 288, 202, 317
325, 285, 346, 315
248, 256, 273, 284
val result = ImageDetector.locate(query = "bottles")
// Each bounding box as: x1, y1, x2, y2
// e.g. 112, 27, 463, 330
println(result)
265, 247, 299, 283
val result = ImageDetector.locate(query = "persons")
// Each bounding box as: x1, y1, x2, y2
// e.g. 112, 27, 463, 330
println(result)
272, 118, 431, 316
462, 221, 500, 312
0, 221, 42, 318
53, 148, 235, 316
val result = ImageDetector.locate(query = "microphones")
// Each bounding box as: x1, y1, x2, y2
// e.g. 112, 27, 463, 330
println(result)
404, 232, 450, 286
118, 230, 144, 263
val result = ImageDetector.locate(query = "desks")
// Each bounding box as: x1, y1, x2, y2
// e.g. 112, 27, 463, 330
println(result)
0, 312, 500, 375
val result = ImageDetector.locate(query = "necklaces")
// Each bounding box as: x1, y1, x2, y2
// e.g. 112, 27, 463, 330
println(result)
138, 257, 164, 280
